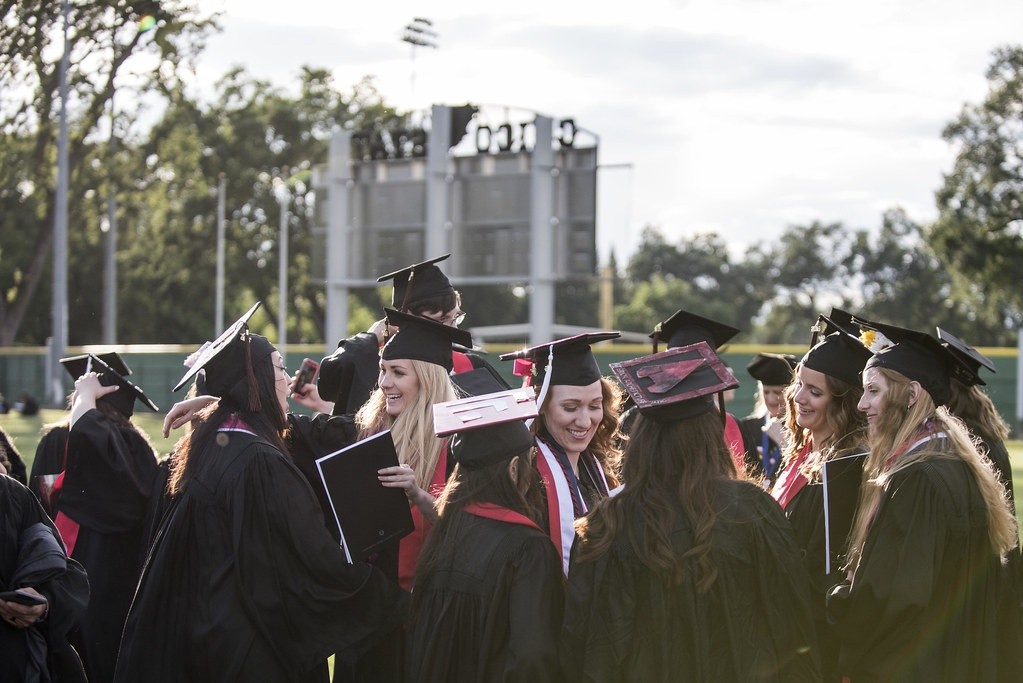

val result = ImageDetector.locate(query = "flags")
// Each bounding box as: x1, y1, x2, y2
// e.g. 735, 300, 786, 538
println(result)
403, 19, 438, 48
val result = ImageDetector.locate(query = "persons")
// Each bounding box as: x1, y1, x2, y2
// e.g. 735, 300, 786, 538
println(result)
0, 252, 1023, 683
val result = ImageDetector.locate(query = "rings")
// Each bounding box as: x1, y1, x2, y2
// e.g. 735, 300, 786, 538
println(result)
10, 616, 16, 622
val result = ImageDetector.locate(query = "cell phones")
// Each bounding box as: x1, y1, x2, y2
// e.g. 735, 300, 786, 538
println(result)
0, 591, 48, 607
291, 358, 319, 398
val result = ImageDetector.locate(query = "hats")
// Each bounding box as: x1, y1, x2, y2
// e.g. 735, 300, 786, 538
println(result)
648, 309, 742, 354
499, 330, 622, 430
379, 307, 473, 374
747, 352, 800, 384
377, 254, 454, 313
609, 341, 741, 428
59, 351, 159, 418
433, 386, 539, 474
802, 307, 997, 410
171, 301, 276, 411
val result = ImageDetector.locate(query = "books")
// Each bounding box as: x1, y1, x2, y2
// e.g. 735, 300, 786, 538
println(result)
822, 453, 867, 574
314, 430, 415, 563
